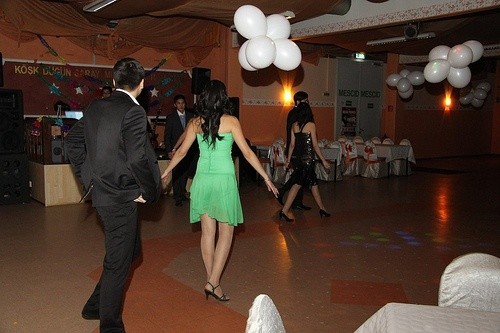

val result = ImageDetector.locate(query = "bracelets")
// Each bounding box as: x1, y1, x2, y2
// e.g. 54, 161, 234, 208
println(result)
264, 177, 271, 182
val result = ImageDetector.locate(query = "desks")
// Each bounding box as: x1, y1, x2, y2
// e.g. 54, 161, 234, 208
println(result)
315, 149, 338, 182
340, 142, 416, 177
355, 302, 500, 333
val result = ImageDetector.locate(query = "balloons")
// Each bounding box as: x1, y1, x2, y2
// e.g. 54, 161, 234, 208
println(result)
383, 39, 492, 109
233, 4, 268, 40
264, 12, 291, 39
237, 40, 259, 71
272, 38, 302, 71
245, 35, 277, 69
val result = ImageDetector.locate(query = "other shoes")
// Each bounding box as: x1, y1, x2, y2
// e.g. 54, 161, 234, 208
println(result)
276, 188, 284, 206
183, 196, 189, 201
176, 199, 183, 207
292, 202, 312, 210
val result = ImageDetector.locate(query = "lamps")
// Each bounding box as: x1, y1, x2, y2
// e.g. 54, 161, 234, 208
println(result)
83, 0, 117, 13
367, 32, 435, 46
404, 22, 423, 40
231, 10, 295, 31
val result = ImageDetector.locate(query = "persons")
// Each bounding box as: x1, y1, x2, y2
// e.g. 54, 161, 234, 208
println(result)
278, 101, 332, 222
60, 57, 158, 333
54, 101, 71, 116
102, 85, 111, 98
159, 78, 282, 303
163, 94, 197, 209
275, 84, 317, 211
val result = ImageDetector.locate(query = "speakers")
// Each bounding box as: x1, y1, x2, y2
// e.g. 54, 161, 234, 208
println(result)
191, 67, 211, 95
0, 87, 25, 154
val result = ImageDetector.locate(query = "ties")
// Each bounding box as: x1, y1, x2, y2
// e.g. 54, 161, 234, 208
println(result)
180, 115, 185, 129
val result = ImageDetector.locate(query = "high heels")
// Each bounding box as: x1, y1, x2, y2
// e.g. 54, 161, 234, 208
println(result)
204, 282, 230, 301
320, 209, 330, 217
280, 210, 295, 222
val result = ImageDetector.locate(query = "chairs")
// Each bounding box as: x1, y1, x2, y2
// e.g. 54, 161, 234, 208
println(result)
438, 253, 500, 313
338, 134, 411, 179
246, 294, 287, 333
272, 137, 340, 184
